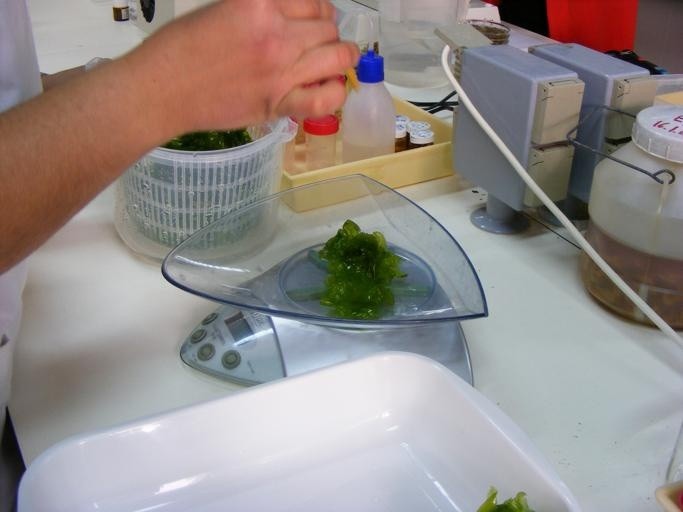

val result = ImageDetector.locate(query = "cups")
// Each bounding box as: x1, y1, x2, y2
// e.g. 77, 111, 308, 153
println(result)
452, 19, 511, 78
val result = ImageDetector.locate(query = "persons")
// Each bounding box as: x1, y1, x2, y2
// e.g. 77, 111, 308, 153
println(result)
0, 1, 362, 511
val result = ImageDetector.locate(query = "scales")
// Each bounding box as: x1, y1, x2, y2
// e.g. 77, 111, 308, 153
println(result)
160, 173, 489, 388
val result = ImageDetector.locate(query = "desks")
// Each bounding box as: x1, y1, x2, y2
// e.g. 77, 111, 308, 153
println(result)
0, 1, 682, 512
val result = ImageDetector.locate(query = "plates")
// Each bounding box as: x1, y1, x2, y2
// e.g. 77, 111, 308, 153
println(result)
11, 352, 580, 512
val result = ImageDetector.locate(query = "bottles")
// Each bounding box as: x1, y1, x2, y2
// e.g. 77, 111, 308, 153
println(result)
392, 113, 434, 153
302, 114, 339, 172
341, 53, 396, 166
284, 114, 297, 168
